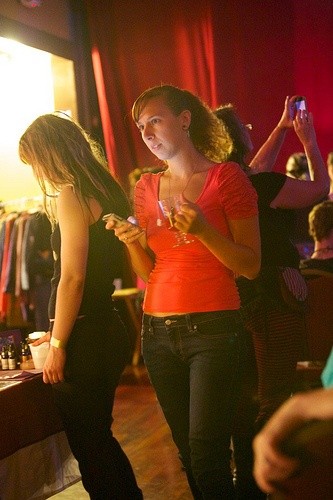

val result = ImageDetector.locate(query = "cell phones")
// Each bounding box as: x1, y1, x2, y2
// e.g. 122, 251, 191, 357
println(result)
295, 96, 308, 119
102, 213, 137, 232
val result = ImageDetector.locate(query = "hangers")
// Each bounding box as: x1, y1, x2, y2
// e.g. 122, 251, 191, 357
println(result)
12, 195, 41, 213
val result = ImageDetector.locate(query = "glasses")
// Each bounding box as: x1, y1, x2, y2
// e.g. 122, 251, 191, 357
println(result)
238, 123, 253, 133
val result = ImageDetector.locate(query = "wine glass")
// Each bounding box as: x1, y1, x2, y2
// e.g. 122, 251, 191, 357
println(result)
160, 196, 197, 248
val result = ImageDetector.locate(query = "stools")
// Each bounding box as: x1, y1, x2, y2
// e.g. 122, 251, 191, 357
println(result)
111, 288, 142, 366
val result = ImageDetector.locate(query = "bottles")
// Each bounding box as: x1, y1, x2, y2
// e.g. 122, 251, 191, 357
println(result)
8, 343, 18, 370
20, 340, 32, 363
0, 345, 9, 370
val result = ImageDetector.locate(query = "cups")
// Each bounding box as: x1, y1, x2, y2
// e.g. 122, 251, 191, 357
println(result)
29, 341, 49, 372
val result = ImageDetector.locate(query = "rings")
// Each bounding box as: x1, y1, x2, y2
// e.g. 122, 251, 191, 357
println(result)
123, 234, 127, 240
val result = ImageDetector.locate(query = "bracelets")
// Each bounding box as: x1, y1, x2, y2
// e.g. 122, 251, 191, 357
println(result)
49, 337, 67, 351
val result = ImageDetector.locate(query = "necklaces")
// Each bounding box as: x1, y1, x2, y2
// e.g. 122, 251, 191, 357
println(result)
314, 246, 332, 254
168, 154, 201, 203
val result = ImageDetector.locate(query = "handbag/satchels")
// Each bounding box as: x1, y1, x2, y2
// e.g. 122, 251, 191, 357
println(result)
278, 263, 310, 313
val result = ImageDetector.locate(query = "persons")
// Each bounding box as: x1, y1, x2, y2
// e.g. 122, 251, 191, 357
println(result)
19, 113, 147, 499
207, 94, 331, 499
298, 200, 333, 284
103, 82, 262, 500
285, 152, 312, 181
325, 152, 333, 202
251, 352, 333, 498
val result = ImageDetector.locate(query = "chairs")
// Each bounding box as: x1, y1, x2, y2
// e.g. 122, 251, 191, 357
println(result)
299, 269, 333, 360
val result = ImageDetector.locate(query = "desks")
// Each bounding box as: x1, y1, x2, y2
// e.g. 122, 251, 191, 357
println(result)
0, 370, 63, 460
